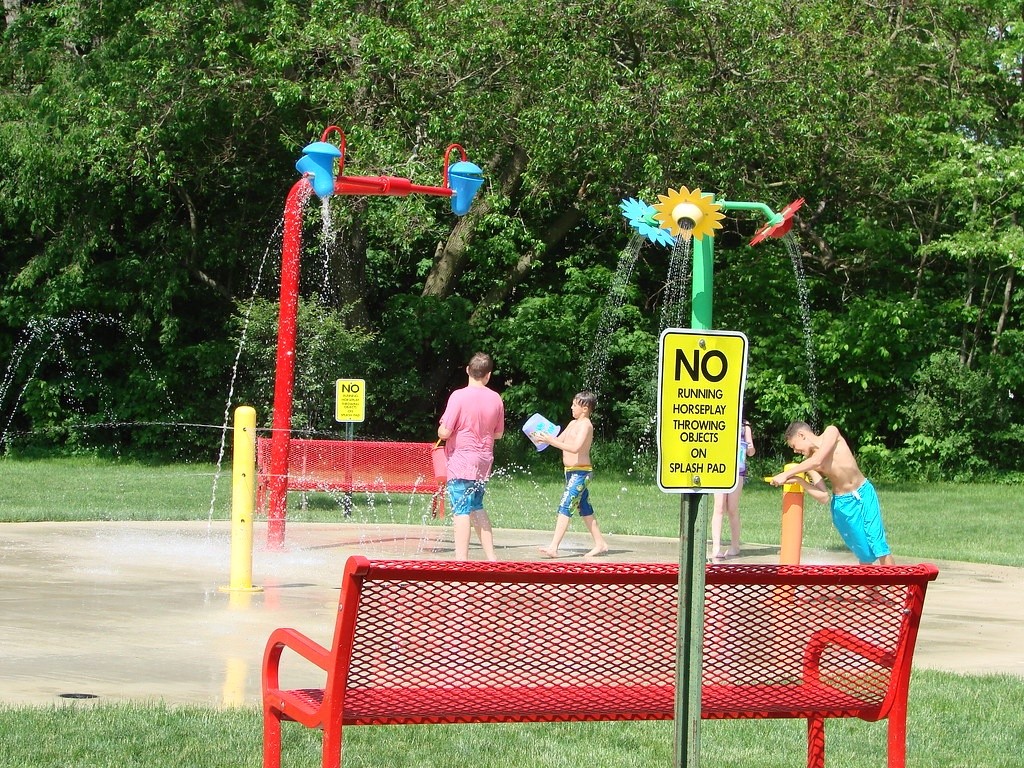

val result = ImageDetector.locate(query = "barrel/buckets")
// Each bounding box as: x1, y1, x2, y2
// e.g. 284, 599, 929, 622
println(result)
522, 413, 561, 452
739, 442, 748, 472
430, 438, 448, 483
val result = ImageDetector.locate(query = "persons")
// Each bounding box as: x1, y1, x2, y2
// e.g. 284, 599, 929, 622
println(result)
535, 391, 608, 557
438, 352, 504, 562
709, 419, 756, 559
769, 421, 904, 606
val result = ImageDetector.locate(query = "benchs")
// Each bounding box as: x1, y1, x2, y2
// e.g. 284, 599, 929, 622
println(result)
263, 555, 940, 768
257, 436, 445, 521
